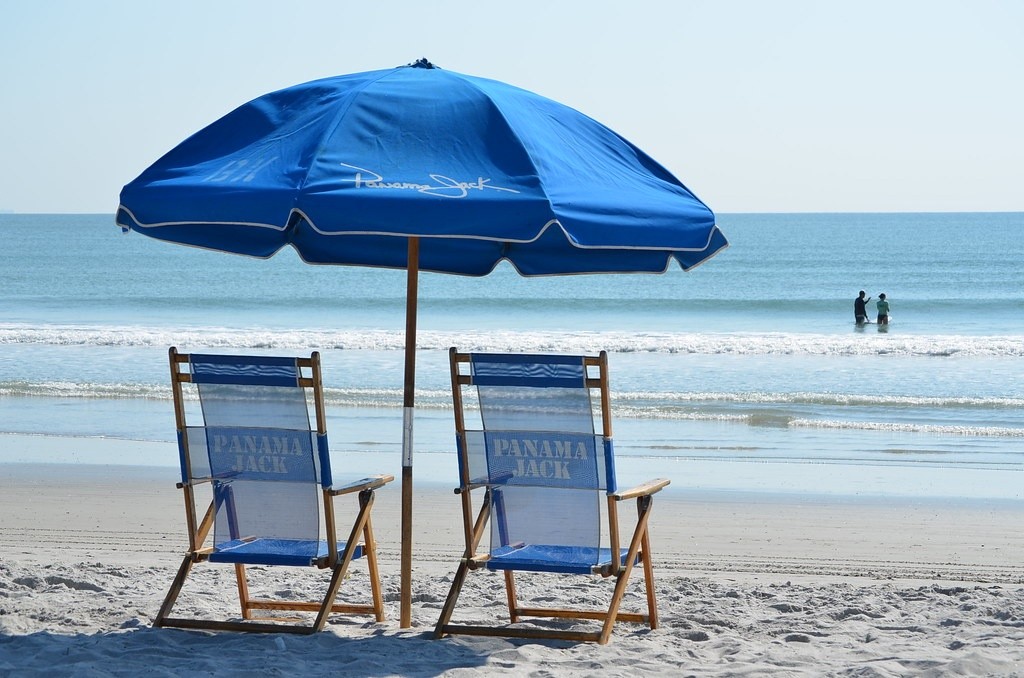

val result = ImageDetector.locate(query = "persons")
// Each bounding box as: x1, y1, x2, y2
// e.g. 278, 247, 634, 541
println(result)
877, 293, 889, 324
854, 290, 871, 325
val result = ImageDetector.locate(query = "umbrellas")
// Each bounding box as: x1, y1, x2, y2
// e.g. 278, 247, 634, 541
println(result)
116, 57, 728, 628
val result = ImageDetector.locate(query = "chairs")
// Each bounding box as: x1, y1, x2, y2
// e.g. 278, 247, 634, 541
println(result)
153, 345, 394, 635
434, 347, 670, 644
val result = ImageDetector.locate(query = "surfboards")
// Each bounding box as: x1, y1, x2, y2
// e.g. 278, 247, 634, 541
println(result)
869, 315, 892, 324
